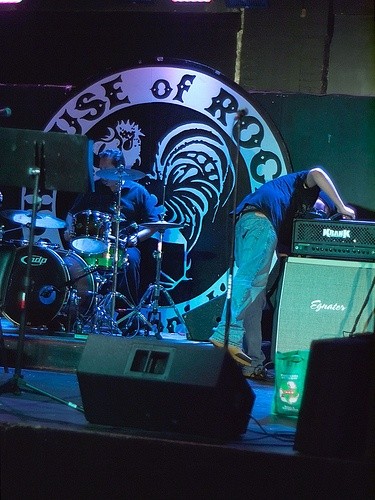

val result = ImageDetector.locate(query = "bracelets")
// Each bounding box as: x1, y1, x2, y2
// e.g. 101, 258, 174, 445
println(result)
134, 235, 140, 243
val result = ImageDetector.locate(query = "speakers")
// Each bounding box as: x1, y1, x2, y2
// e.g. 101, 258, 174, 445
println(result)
183, 290, 227, 341
76, 333, 257, 439
276, 257, 375, 462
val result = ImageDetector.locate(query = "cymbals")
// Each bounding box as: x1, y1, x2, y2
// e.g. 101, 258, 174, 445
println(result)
140, 221, 181, 229
0, 210, 67, 229
96, 168, 146, 181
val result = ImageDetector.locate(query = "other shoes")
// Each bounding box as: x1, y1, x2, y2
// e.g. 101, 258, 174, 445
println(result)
210, 337, 252, 367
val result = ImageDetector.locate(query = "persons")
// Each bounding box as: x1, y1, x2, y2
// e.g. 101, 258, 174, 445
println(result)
209, 168, 356, 378
70, 148, 159, 305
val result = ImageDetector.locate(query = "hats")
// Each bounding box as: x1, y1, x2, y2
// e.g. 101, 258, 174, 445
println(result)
318, 190, 336, 213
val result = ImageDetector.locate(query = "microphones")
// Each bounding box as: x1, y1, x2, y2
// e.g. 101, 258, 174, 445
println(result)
234, 107, 249, 120
41, 291, 52, 298
0, 107, 12, 117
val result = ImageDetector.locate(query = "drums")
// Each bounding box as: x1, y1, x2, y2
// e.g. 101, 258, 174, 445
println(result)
2, 244, 95, 330
70, 210, 112, 255
81, 243, 126, 270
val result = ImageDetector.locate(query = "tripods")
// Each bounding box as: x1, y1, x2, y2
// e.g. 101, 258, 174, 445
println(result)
0, 128, 193, 414
